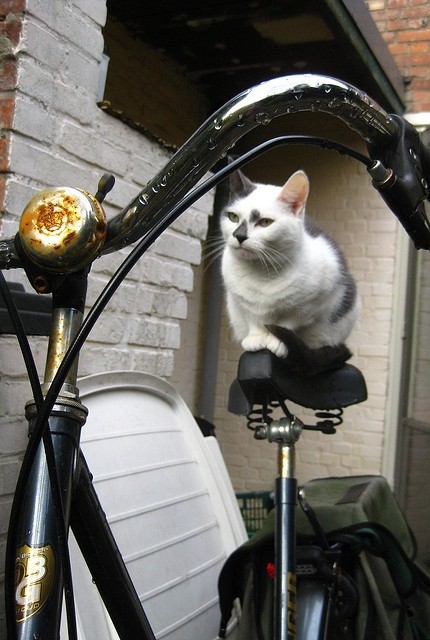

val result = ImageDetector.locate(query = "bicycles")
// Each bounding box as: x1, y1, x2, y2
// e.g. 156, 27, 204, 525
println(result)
0, 72, 430, 638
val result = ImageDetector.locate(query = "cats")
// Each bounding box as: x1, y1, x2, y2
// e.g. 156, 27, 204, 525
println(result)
201, 156, 361, 359
